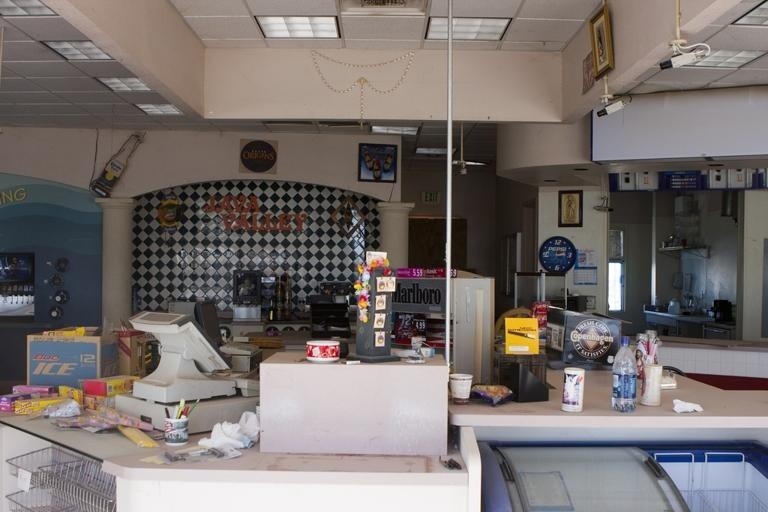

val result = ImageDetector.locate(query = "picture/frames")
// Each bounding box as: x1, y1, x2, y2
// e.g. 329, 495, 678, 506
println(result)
233, 269, 262, 304
558, 189, 583, 227
357, 142, 396, 183
589, 5, 614, 80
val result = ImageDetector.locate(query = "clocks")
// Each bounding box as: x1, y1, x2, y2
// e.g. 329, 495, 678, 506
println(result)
539, 236, 576, 273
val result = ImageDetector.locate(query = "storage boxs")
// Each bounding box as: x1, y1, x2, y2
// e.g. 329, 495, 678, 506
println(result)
27, 334, 118, 387
111, 328, 146, 375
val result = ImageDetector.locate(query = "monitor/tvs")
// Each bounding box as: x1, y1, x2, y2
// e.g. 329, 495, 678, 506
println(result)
193, 300, 224, 349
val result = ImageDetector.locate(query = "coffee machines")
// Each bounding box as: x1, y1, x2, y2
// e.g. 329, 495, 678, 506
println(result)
711, 299, 733, 324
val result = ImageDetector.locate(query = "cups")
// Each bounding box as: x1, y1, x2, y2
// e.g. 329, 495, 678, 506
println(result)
163, 417, 190, 447
641, 364, 663, 407
421, 347, 435, 358
449, 373, 474, 405
560, 367, 585, 413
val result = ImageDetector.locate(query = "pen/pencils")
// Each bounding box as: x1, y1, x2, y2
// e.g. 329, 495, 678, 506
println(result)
166, 399, 199, 419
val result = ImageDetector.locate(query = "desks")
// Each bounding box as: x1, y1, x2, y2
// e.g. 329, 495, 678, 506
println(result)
449, 364, 768, 512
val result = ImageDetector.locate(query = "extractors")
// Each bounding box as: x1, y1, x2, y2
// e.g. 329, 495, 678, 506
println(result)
658, 195, 710, 258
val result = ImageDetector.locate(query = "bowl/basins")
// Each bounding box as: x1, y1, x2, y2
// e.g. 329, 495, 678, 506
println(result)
304, 340, 342, 363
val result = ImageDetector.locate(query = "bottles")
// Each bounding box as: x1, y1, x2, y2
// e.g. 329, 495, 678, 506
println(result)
330, 337, 349, 358
611, 335, 638, 412
635, 333, 649, 380
645, 329, 658, 365
268, 297, 275, 321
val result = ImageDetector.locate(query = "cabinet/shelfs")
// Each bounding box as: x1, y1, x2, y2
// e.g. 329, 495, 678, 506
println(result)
391, 267, 495, 386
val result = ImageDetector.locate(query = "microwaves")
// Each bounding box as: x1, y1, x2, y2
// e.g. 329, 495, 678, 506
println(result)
546, 294, 588, 314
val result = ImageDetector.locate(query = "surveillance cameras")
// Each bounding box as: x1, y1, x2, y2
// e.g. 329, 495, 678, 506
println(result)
657, 37, 713, 73
596, 89, 633, 120
